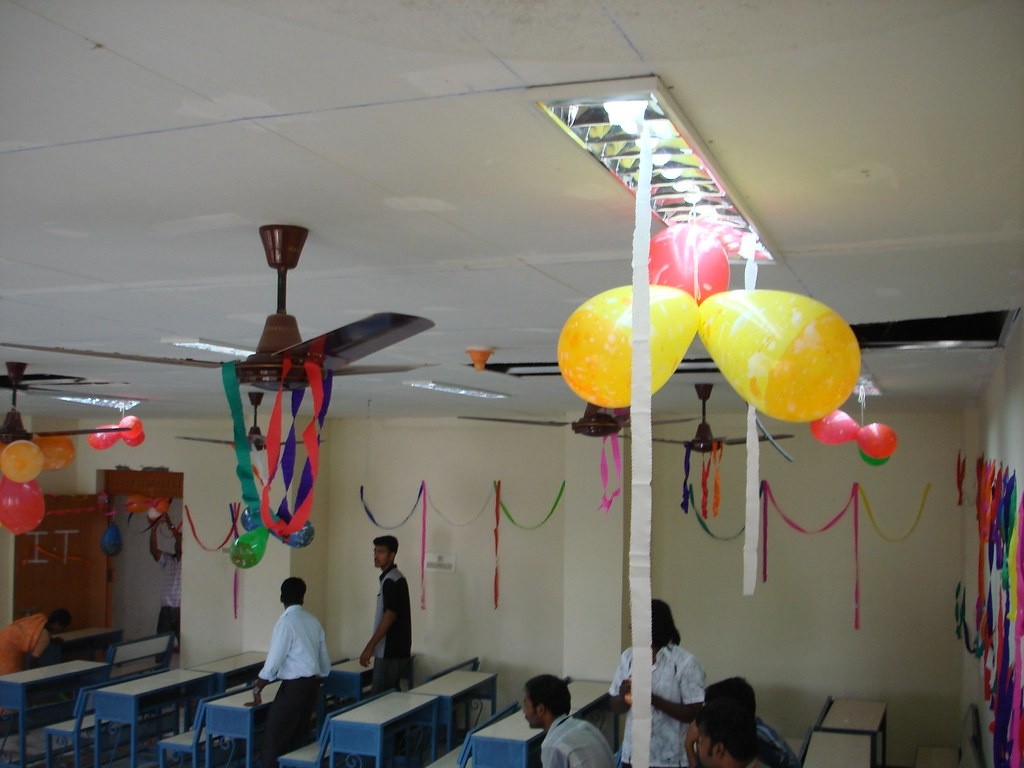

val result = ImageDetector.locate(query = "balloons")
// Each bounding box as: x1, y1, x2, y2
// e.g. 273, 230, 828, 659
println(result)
230, 502, 315, 569
558, 224, 898, 465
0, 435, 75, 535
127, 494, 170, 523
88, 416, 146, 450
102, 522, 124, 556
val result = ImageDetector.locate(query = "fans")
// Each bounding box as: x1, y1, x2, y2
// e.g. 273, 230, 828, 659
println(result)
0, 224, 440, 391
173, 392, 325, 451
458, 401, 704, 437
652, 384, 794, 452
0, 362, 131, 444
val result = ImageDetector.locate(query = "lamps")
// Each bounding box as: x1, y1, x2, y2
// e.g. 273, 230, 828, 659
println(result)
525, 75, 778, 268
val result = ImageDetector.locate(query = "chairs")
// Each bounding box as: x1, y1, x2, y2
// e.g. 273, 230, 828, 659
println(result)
915, 703, 984, 768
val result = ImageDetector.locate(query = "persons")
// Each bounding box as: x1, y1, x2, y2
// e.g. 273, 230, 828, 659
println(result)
609, 600, 706, 768
359, 535, 412, 696
0, 607, 71, 719
148, 516, 183, 672
684, 676, 804, 768
522, 675, 618, 768
244, 578, 332, 768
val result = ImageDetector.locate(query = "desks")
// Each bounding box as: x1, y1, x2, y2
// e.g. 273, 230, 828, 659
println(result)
323, 654, 416, 731
0, 660, 110, 768
52, 627, 124, 689
567, 680, 620, 754
94, 670, 216, 768
820, 700, 888, 768
185, 652, 268, 734
409, 669, 498, 764
803, 731, 874, 768
470, 710, 577, 768
205, 680, 326, 768
329, 691, 439, 768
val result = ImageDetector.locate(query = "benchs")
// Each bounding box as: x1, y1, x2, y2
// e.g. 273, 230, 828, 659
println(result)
776, 695, 832, 768
43, 632, 571, 768
